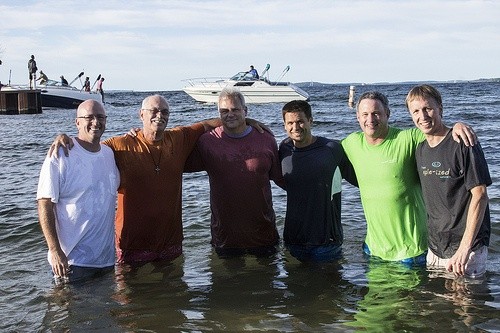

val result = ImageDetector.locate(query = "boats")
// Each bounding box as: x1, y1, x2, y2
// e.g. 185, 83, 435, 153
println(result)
0, 72, 102, 110
180, 63, 311, 105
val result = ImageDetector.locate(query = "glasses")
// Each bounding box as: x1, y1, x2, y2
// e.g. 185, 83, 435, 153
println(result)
143, 108, 172, 116
77, 115, 108, 121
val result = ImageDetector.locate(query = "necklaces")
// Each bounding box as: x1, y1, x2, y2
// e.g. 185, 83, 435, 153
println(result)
144, 142, 161, 175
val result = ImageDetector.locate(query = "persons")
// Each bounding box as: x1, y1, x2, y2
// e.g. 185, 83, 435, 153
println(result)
340, 91, 478, 264
36, 70, 47, 85
97, 78, 105, 103
50, 95, 275, 264
123, 91, 286, 248
60, 76, 68, 86
37, 99, 120, 281
278, 100, 359, 261
28, 55, 37, 88
406, 85, 492, 278
83, 77, 90, 92
249, 65, 259, 79
